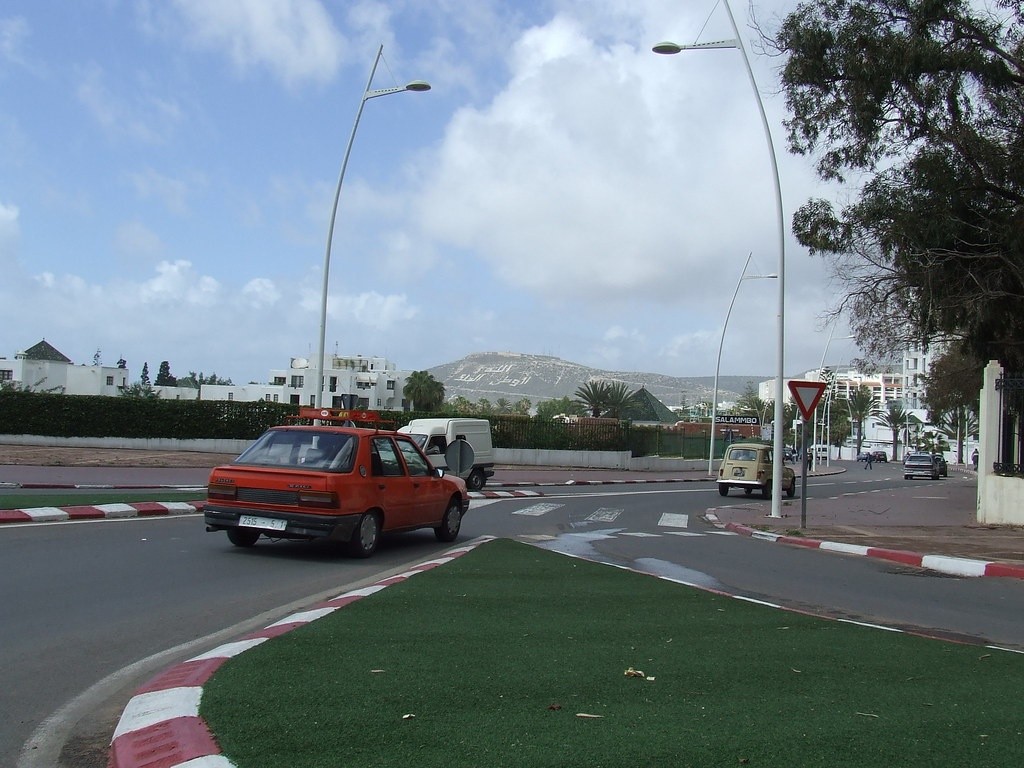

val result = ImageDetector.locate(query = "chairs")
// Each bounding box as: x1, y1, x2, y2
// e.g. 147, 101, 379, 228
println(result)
303, 447, 323, 465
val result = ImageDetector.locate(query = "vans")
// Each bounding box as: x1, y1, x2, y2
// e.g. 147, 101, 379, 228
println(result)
376, 416, 496, 490
810, 444, 829, 459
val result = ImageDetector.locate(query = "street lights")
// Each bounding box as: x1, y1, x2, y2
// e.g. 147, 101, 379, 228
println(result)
708, 252, 778, 476
807, 324, 859, 472
652, 0, 788, 522
309, 44, 431, 452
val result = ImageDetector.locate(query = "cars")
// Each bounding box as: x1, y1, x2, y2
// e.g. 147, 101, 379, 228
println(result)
201, 406, 473, 561
716, 443, 796, 500
783, 446, 793, 461
857, 451, 871, 461
901, 450, 949, 477
870, 450, 888, 462
903, 454, 941, 480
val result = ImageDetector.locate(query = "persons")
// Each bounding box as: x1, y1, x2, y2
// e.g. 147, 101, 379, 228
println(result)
972, 449, 979, 466
738, 451, 751, 460
807, 448, 813, 471
429, 439, 440, 453
864, 452, 872, 470
782, 449, 786, 466
792, 448, 796, 454
